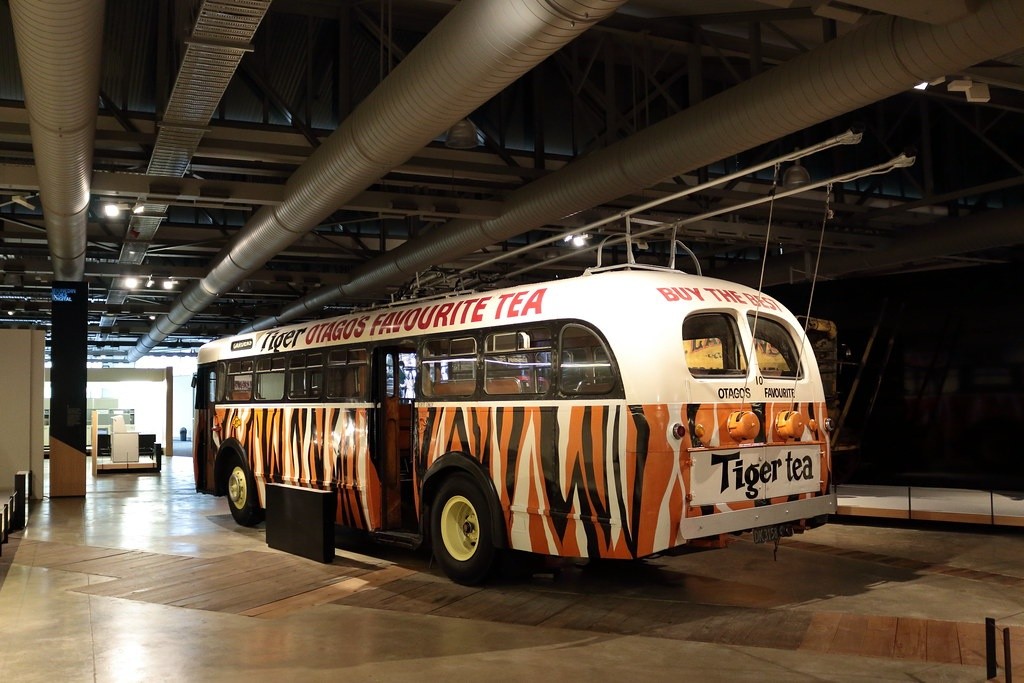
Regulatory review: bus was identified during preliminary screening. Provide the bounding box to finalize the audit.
[190,268,836,587]
